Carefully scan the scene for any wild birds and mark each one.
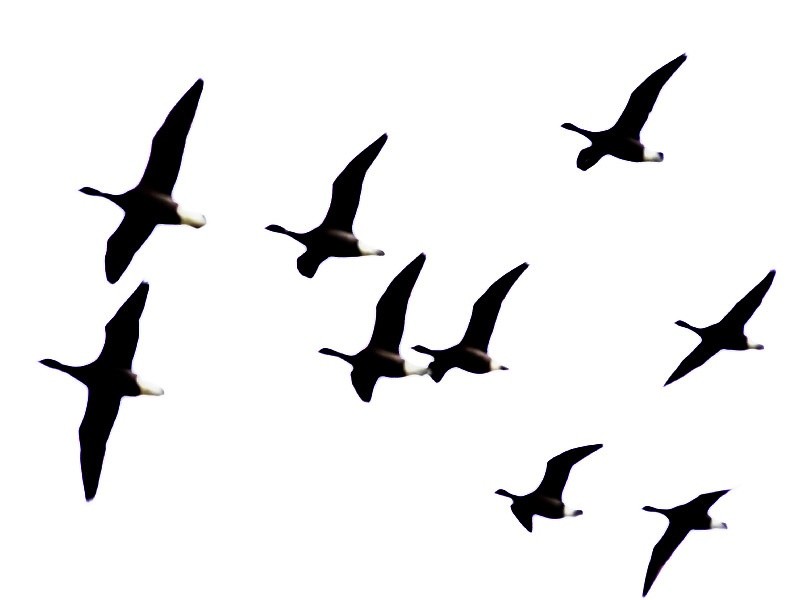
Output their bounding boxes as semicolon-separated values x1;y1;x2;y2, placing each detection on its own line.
316;252;427;401
78;77;206;283
495;444;603;531
665;269;778;385
644;490;728;597
265;134;388;280
562;52;689;171
33;283;162;502
412;260;530;382
